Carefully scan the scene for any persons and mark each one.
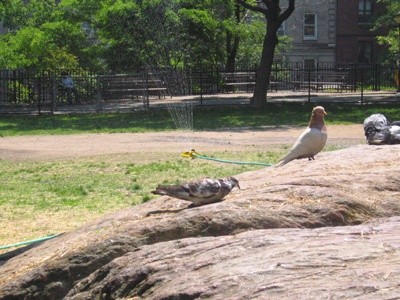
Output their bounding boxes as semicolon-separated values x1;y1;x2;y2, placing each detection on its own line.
57;70;84;107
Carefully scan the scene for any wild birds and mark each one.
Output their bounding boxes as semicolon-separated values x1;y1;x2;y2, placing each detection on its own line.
363;112;400;145
275;106;328;168
60;72;76;91
151;177;241;208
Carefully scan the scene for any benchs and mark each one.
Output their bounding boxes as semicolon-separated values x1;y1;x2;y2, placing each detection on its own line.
105;79;166;96
292;73;348;91
220;71;278;93
